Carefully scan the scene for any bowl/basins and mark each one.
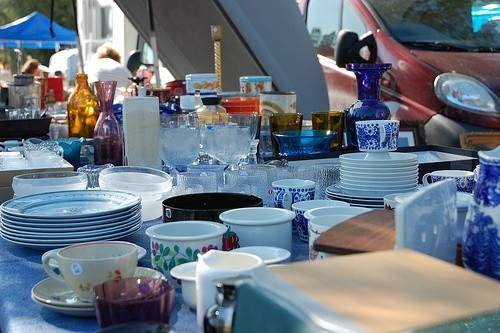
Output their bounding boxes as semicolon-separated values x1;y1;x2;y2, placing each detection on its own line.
12;171;88;193
99;166;172;224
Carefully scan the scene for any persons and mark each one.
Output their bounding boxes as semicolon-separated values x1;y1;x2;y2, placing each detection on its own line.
0;60;11;80
82;43;134;97
136;65;153;87
23;60;50;80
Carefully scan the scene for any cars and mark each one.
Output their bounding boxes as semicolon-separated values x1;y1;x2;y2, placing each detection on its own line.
295;0;500;152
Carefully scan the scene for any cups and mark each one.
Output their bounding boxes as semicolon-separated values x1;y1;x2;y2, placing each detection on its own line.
383;193;416;211
40;240;138;302
456;192;472;245
422;170;474;194
24;73;372;332
356;119;399;153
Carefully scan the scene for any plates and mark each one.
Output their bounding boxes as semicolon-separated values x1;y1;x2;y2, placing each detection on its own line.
32;290;172;317
0;192;142;252
31;267;165;308
323;147;421;212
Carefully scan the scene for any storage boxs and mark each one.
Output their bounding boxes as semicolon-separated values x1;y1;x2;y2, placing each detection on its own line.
266;142;478;183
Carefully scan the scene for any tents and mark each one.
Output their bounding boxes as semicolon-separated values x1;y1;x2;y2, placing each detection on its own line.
0;12;81;76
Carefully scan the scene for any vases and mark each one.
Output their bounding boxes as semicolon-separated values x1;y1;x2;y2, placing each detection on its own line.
344;62;393;146
92;80;122;164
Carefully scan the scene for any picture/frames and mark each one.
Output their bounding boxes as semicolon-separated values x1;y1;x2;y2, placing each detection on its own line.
398;121;424;146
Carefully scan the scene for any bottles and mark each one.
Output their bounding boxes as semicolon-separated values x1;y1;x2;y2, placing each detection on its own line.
9;71;122;166
462;146;500;281
346;62;393;147
197;96;227;124
